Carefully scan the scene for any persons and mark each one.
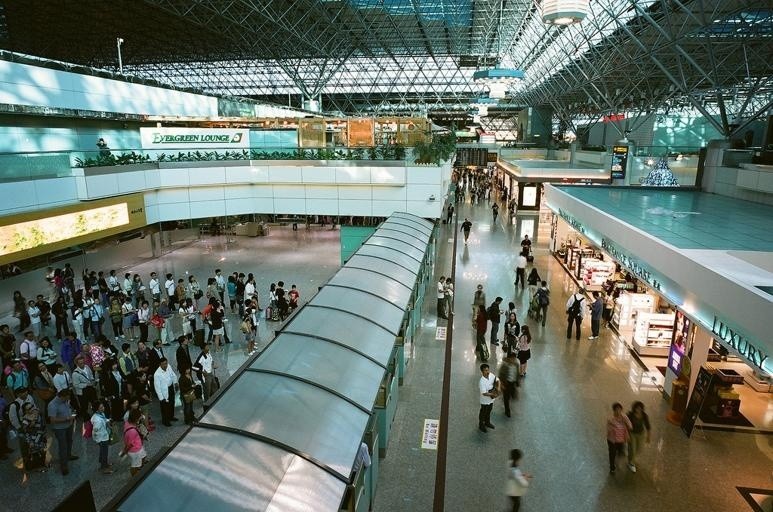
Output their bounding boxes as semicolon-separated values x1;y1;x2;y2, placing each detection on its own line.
606;402;634;475
96;138;111;157
503;449;534;512
292;215;364;231
626;401;652;473
211;217;221;236
389;132;398;144
674;311;687;353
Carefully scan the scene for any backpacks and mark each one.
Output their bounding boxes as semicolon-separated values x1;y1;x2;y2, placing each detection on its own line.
487;305;498;319
476;336;489;360
539;290;549;306
568;294;584;316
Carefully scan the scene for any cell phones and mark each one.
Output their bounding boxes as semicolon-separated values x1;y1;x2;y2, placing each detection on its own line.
71;413;77;417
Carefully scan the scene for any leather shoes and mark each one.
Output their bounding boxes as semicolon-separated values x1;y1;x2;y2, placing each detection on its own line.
162;416;179;426
480;425;487;432
70;456;79;460
486;422;494;429
505;412;511;417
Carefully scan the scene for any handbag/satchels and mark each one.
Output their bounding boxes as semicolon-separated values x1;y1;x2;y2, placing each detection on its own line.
489;377;501;399
82;419;93;439
78;386;97;401
240;316;252;333
2;369;55;421
109;426;120;445
505;467;527;497
194;290;203;299
99;311;142;326
184;389;196;403
182;316;192;336
25;448;46;470
151;316;165;328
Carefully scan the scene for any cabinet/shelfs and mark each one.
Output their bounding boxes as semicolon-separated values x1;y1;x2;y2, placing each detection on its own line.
564;246;676;355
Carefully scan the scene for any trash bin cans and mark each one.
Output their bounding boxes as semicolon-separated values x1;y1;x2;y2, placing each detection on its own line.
666;377;689;426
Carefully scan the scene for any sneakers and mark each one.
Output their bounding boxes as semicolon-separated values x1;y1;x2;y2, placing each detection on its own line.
589;336;598;340
491;339;499;346
207;340;232;353
115;335;139;342
628;463;637;472
247;342;259;356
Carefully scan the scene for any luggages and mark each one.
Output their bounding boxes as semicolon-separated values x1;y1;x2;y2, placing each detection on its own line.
266;306;281;321
527;251;534;262
194;322;205;345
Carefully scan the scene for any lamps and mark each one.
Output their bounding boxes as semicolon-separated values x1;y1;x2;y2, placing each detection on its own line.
541;0;588;25
465;1;525;133
